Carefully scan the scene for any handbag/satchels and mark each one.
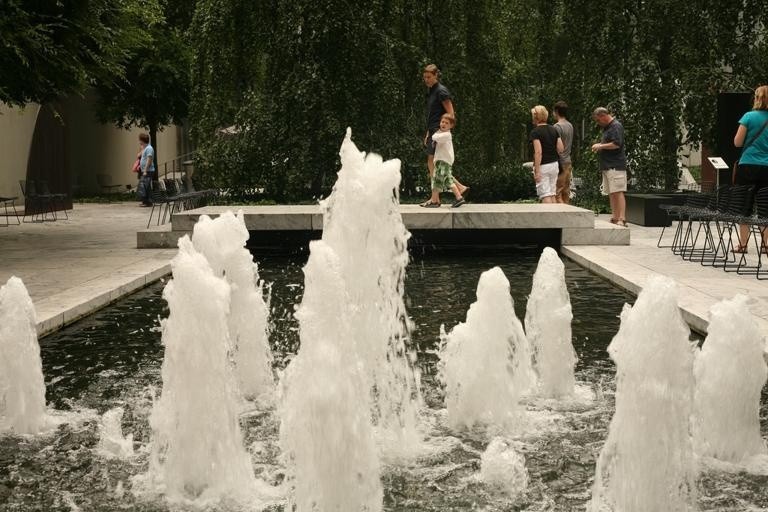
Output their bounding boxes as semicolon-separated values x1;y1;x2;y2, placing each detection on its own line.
137;176;150;198
133;160;140;171
731;160;739;186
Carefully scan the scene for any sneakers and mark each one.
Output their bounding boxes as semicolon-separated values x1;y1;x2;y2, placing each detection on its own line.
420;187;470;207
140;203;152;207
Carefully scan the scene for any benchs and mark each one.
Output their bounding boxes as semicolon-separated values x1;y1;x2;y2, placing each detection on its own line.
619;192;688;228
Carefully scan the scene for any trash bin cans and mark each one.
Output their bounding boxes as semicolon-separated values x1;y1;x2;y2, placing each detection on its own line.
182;160;196;193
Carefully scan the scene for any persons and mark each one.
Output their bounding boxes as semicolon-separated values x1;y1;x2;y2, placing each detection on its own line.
731;85;768;253
530;104;565;203
591;107;627;227
550;100;574;205
423;113;466;208
137;133;156;207
137;143;155;203
419;63;471;206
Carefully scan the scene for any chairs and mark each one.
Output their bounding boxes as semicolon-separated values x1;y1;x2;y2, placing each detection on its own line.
94;171;124;204
0;176;71;229
143;172;222;236
651;179;767;286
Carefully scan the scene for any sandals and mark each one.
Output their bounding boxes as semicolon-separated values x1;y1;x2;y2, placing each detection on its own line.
731;244;747;253
